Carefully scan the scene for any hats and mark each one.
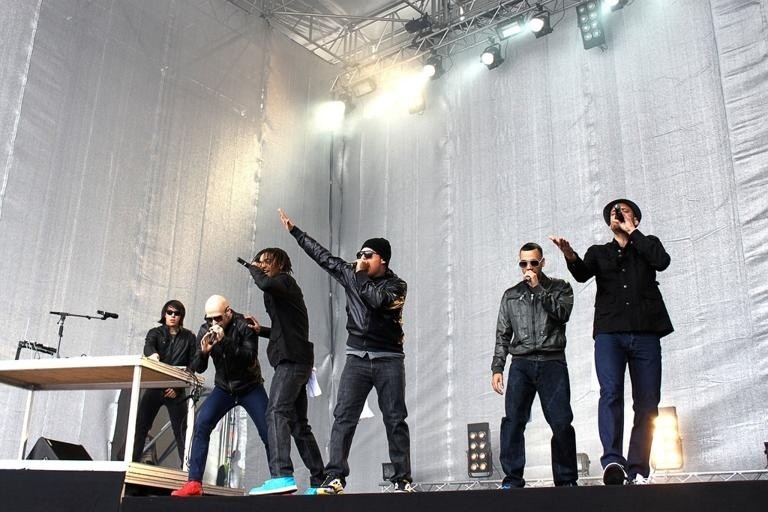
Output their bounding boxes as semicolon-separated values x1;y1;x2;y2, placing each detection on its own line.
362;236;392;263
602;198;643;228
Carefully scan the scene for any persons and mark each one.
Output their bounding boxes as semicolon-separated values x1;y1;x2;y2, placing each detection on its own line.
547;199;669;486
277;206;412;495
129;299;196;471
247;247;325;497
490;242;577;488
169;295;269;497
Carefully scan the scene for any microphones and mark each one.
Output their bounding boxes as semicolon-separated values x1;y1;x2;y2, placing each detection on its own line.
344;260;368;269
613;203;627;225
236;256;263;272
97;309;118;318
208;323;222;345
524;271;534;282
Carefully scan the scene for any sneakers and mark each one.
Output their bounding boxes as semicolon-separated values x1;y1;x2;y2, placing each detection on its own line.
600;463;627;487
303;486;318;496
502;482;517;489
170;480;204;498
315;471;345;494
626;472;651;484
391;478;413;493
247;476;299;496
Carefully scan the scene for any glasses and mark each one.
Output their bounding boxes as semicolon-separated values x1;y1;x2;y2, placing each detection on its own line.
518;257;545;268
166;309;183;317
356;250;378;260
203;313;226;323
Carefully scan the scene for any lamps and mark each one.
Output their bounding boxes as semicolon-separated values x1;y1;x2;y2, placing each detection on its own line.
602;461;630;486
575;1;605;51
336;93;357;118
530;11;553;38
423;57;445;82
610;0;630;13
576;454;591;478
467;422;493;477
653;406;685;471
494;16;527;42
382;462;397;481
0;355;203;507
351;80;377;98
400;70;427;117
480;43;505;72
